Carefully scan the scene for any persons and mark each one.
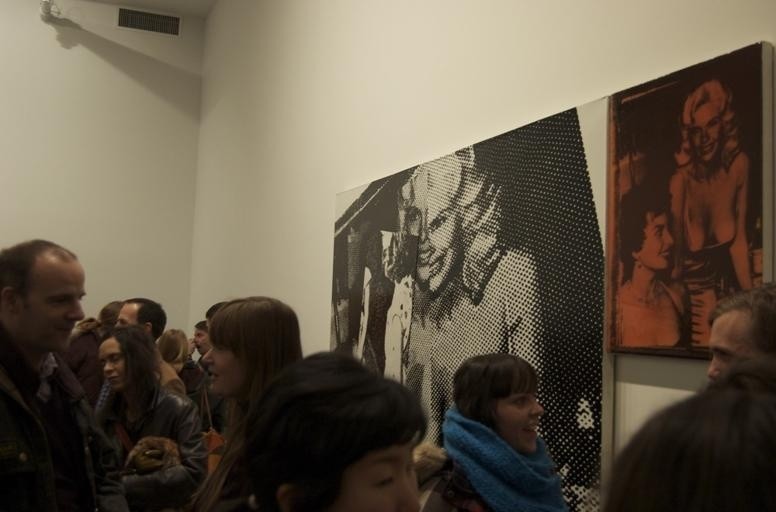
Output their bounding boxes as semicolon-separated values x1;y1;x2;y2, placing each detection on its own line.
1;239;426;512
614;173;690;348
600;352;776;509
707;283;775;383
669;78;754;299
431;142;548;453
382;163;430;462
417;353;572;512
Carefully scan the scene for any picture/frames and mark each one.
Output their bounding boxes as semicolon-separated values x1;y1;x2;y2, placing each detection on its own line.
607;40;772;360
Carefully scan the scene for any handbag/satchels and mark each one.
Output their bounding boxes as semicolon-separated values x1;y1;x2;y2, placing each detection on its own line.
203;427;225;476
